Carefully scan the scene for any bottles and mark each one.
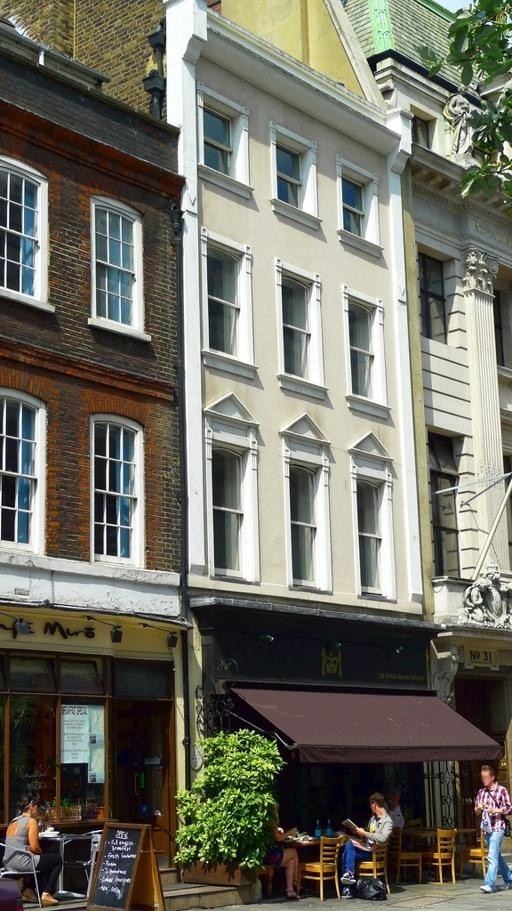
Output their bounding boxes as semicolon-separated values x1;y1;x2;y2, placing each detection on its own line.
326;819;334;837
314;818;322;840
38;819;44;832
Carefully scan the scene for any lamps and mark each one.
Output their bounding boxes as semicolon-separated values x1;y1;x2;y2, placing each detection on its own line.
81;614;123;644
1;612;30;636
138;623;178;649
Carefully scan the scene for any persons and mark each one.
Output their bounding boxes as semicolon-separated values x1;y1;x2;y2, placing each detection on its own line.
466;577;489;622
261;826;306;901
383;792;406;885
339;792;394;901
460;564;508;627
472;764;512;894
2;790;64;908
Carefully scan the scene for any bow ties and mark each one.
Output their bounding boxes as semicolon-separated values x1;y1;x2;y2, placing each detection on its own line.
374;815;381;820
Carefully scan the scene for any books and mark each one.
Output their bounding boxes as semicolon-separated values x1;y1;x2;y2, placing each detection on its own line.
341;819;358;833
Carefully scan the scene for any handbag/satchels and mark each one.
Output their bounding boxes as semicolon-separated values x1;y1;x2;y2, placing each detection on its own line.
504;818;511;837
352;878;387;900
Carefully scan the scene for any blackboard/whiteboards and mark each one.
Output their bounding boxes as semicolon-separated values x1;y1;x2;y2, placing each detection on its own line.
86;822;148;911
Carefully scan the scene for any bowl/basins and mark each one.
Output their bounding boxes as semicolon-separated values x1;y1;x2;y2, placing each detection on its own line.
38;827;60;837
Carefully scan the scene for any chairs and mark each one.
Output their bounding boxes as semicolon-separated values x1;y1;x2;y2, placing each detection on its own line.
0;843;46;909
258;819;497;903
62;830;104;893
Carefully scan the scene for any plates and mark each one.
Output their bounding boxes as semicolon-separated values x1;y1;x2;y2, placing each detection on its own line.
282;830;313;844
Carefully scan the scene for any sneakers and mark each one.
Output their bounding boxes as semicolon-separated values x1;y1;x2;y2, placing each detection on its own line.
341;871;356;885
342;886;352;898
480;884;497;893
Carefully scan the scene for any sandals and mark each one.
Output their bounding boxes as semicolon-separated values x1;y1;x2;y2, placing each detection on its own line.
285;889;303;899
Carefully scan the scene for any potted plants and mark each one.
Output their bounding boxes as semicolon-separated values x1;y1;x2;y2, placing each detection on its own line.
43;797;84;823
175;729;284;887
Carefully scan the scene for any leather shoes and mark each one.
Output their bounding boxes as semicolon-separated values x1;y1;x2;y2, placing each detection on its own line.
22;896;39;903
40;896;59;907
